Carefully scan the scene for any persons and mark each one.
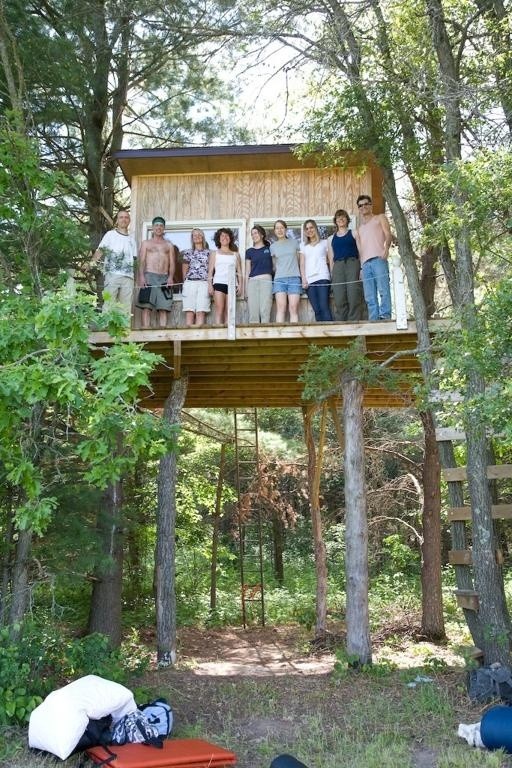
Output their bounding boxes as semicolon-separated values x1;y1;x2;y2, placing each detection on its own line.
83;210;137;331
136;217;175;329
182;228;210;325
327;209;363;321
269;219;304;323
298;220;332;322
244;225;273;323
355;195;391;320
208;227;242;324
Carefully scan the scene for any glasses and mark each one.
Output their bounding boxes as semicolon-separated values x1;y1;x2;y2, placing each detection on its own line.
359;202;369;208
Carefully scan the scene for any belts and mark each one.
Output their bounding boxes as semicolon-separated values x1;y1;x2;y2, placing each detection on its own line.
185;277;206;281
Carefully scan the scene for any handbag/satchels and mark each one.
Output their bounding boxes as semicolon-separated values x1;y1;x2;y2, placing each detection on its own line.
142;698;173;736
28;714;112;761
